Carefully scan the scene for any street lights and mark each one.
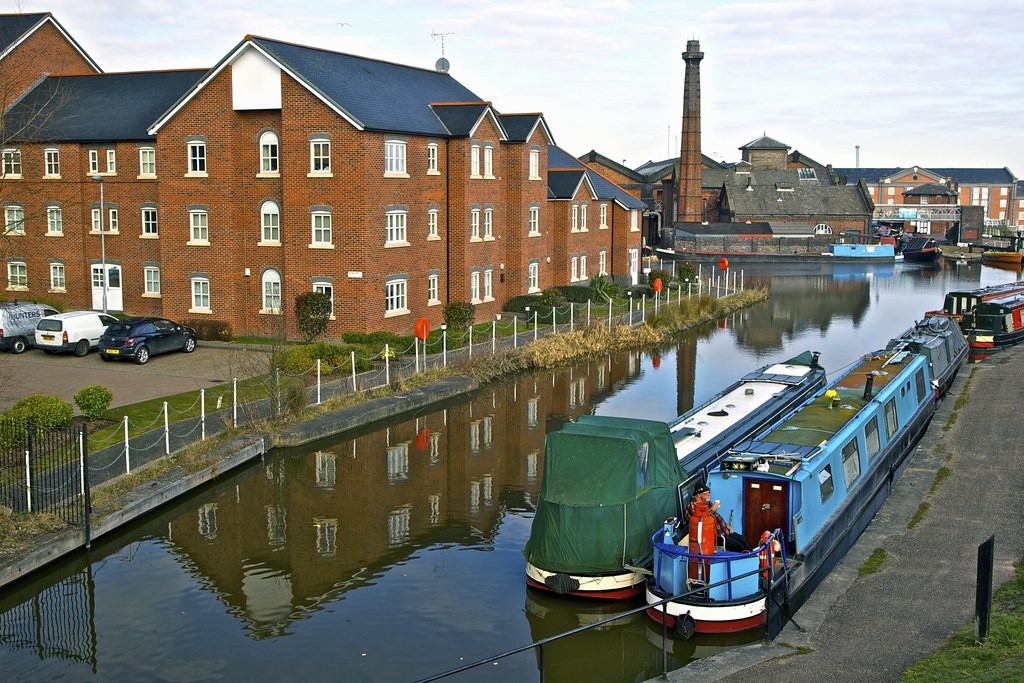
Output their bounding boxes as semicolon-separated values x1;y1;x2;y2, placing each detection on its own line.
91;175;108;319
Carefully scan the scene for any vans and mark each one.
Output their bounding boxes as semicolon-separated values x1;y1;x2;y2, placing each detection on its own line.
34;310;120;357
0;299;62;354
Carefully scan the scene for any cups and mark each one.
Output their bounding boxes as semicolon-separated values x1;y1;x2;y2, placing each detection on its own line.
715;500;720;508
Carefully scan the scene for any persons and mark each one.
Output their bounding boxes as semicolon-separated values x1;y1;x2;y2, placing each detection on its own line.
685;482;752;552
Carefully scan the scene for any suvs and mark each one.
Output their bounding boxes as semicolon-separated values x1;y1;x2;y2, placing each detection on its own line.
97;316;198;365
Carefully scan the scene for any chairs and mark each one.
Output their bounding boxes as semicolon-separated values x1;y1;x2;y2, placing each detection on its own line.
685;501;727;553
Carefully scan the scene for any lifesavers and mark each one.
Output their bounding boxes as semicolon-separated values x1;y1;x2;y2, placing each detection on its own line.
759;529;777;579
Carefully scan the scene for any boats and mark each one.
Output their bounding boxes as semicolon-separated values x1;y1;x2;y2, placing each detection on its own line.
983;250;1022;263
903;238;941;261
645;349;935;639
522;349;828;602
886;280;1024;397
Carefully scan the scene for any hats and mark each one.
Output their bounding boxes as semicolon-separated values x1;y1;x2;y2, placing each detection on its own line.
693;484;709;495
